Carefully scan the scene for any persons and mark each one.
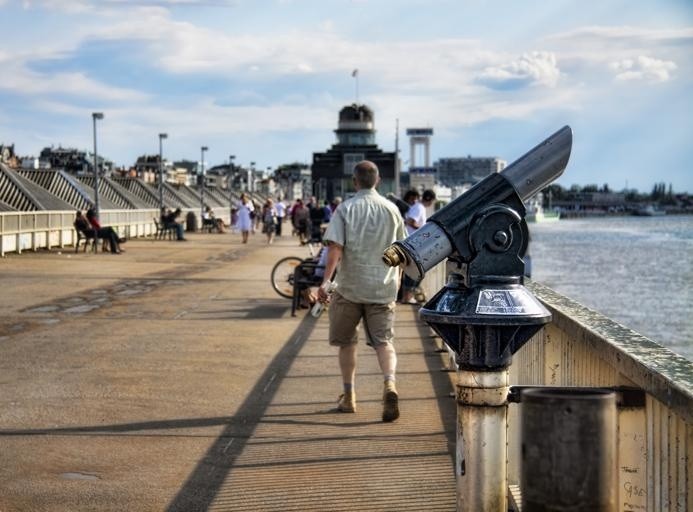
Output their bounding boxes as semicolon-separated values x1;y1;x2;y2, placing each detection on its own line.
154;184;437;313
74;210;126;254
85;207;126;252
316;159;410;423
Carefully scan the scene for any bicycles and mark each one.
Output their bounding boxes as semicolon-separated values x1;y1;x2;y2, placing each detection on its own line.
271;254;311;298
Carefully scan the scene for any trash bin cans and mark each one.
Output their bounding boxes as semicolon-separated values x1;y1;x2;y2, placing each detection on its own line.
186;212;196;232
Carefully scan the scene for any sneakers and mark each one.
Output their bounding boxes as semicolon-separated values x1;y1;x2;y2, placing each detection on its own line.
401;298;420;305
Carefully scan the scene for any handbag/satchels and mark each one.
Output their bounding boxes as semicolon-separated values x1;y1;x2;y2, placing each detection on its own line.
299;218;306;225
271;217;278;224
249;211;256;219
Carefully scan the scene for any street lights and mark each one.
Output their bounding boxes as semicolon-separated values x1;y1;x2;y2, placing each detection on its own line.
249;161;254;202
200;147;209;229
158;133;167;213
228;155;235;207
91;112;103;223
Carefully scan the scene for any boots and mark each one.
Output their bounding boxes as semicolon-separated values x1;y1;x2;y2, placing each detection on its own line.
337;389;356;413
382;380;400;422
